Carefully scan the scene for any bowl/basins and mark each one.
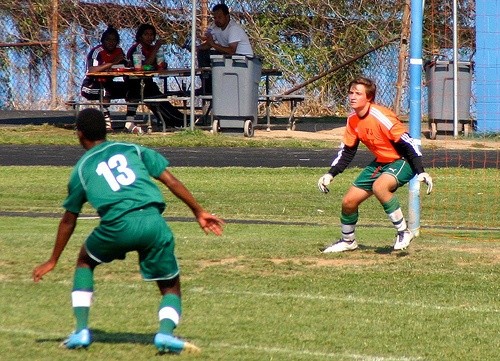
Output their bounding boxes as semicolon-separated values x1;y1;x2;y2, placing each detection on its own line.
111;65;124;71
143;65;154;71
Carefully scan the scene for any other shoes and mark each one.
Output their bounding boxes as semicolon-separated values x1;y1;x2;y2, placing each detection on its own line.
124;123;143;133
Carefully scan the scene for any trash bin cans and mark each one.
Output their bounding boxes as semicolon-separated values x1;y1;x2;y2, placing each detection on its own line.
209;53;264;137
423;60;473;140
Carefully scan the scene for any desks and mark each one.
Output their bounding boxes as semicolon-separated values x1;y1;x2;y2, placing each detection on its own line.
85;67;283;134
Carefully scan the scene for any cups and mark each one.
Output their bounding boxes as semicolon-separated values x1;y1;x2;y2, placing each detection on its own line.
133;52;142;70
156;52;165;70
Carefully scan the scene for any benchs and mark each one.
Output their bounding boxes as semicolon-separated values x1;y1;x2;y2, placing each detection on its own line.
63;89;306;132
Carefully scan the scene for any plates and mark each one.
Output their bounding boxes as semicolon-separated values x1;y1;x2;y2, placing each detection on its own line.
124;67;134;71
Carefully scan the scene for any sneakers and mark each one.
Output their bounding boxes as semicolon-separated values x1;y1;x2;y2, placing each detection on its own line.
394;228;414;251
324;239;357;253
61;329;90;348
154;333;201;355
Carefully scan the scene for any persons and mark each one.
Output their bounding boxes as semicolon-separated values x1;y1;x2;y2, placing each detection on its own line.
81;29;144;134
197;3;254;96
32;108;226;355
318;77;433;253
125;23;199;128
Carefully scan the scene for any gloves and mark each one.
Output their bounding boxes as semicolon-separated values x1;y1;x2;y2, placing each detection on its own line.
318;174;334;194
417;173;432;195
